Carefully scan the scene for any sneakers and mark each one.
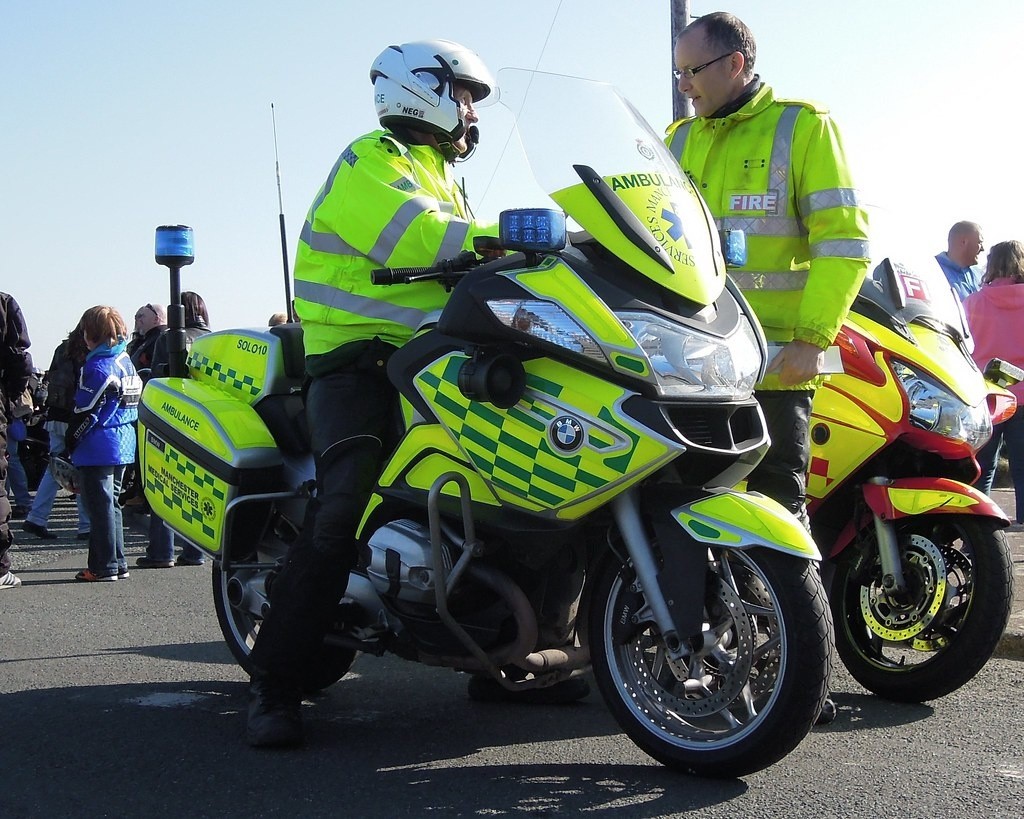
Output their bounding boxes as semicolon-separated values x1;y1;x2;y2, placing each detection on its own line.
245;663;303;749
74;562;130;582
0;571;21;588
135;549;203;567
468;656;590;706
22;519;60;542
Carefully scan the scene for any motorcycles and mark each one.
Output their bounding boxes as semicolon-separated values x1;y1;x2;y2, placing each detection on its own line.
807;258;1024;701
131;66;837;780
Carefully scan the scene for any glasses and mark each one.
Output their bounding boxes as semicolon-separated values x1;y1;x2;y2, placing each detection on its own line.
674;53;731;80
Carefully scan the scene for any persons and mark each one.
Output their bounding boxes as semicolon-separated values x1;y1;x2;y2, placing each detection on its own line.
935;223;984;299
0;276;210;590
663;12;870;723
244;39;588;749
962;242;1024;522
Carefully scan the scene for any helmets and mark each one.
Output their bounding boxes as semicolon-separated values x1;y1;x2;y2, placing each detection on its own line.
371;41;492;139
